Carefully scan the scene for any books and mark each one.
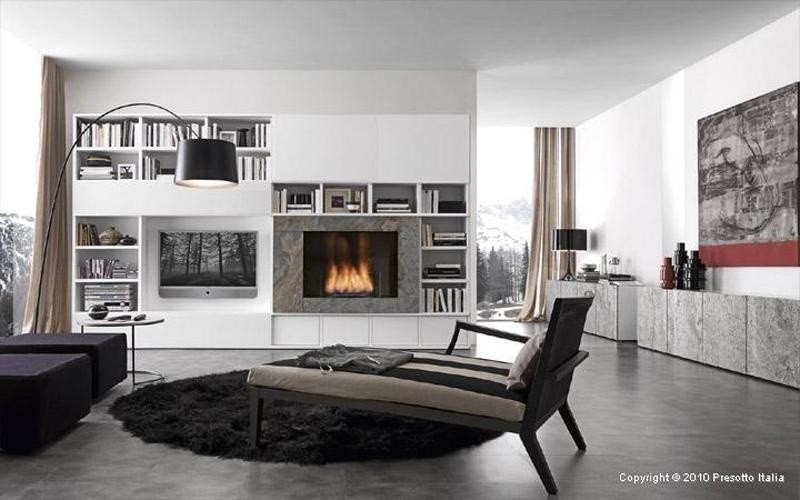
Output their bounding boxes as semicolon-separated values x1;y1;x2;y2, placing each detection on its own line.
272;182;466;215
578;270;645;288
74;117;269;184
77;221;137;311
421;223;468;314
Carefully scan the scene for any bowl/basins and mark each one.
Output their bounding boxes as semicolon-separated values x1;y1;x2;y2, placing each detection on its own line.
580;264;597;272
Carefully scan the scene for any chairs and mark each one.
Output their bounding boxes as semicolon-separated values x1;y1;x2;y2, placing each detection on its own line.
245;291;594;496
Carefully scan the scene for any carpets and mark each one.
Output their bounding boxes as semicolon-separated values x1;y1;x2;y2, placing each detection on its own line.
105;358;506;467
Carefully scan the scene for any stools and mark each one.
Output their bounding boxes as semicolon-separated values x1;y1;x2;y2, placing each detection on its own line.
1;333;128;456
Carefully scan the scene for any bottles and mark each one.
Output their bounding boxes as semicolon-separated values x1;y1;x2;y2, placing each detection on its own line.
600;254;607;279
609;257;620;274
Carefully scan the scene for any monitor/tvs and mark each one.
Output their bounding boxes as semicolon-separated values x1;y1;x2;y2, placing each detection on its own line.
158;229;257;298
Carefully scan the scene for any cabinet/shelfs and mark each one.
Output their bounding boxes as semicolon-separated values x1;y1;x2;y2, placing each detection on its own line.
544;278;800;391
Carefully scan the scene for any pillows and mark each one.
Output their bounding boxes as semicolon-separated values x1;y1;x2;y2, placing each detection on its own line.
504;329;548;393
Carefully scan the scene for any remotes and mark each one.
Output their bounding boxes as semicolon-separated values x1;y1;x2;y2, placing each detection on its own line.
135;314;145;320
109;315;131;321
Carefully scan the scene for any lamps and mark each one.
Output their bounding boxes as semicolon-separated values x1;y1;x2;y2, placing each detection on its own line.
551;228;587;281
33;102;238;333
